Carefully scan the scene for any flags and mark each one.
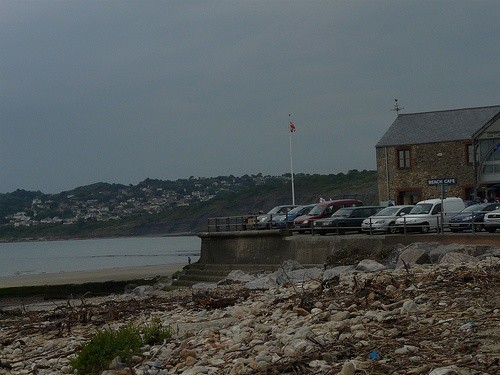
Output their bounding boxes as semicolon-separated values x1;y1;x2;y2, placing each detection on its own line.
290;122;296;133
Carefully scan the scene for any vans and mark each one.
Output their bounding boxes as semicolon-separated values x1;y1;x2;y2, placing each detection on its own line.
395;196;466;233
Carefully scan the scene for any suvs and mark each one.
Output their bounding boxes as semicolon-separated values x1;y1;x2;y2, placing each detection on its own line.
293;198;364;234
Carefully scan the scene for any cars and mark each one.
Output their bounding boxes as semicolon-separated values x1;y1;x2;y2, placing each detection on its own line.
448;202;500;232
252;204;300;230
483;207;500;233
313;206;387;235
276;204;316;229
361;204;415;234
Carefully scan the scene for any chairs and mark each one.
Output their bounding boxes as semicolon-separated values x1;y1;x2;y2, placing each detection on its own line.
347;211;356;217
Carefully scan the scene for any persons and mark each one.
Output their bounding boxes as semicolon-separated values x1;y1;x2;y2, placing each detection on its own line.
479;195;500;203
